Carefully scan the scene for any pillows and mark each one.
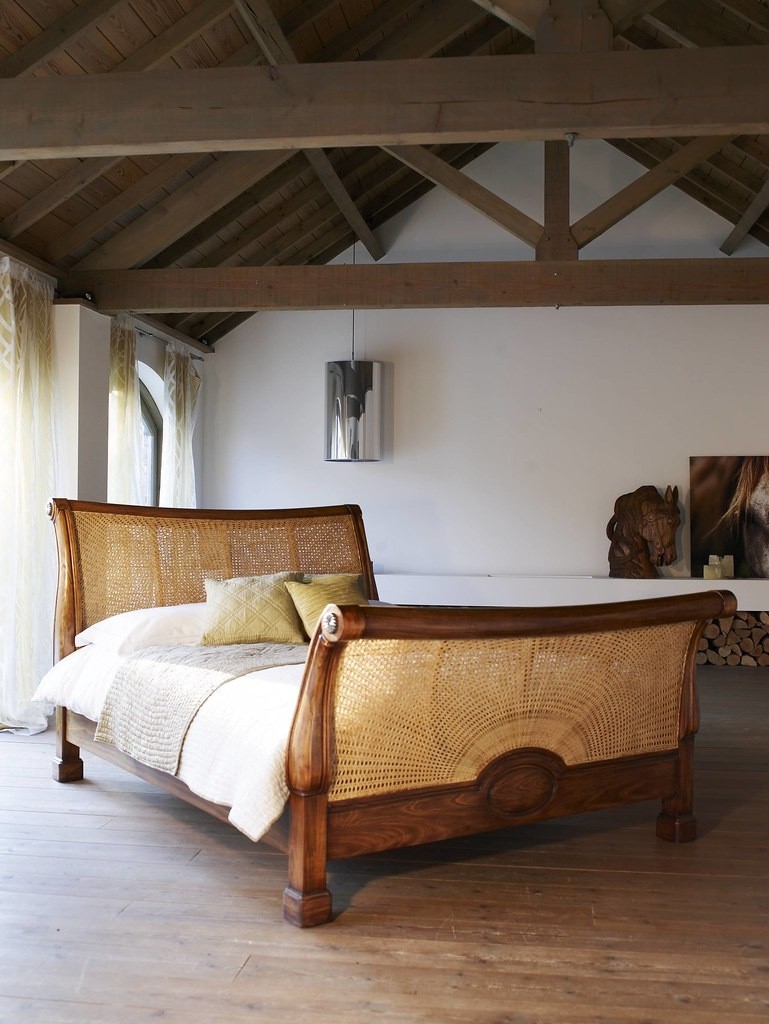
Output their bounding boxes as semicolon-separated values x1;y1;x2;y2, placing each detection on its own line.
75;602;206;661
282;574;370;638
202;570;306;645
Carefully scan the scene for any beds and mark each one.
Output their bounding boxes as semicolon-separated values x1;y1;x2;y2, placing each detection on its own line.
29;497;739;930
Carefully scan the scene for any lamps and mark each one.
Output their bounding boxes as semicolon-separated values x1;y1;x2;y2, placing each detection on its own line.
322;237;379;462
